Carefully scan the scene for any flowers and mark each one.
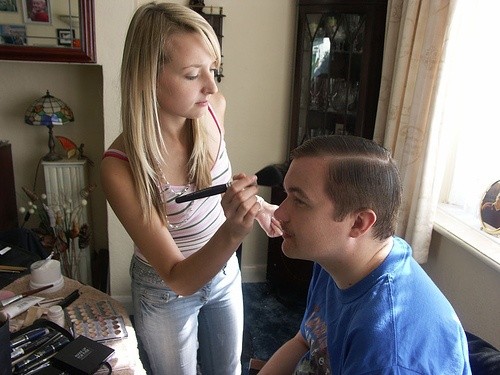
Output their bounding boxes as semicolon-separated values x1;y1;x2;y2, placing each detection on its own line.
12;187;92;250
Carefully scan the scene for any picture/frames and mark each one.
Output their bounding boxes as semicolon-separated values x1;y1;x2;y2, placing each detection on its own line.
0;0;97;64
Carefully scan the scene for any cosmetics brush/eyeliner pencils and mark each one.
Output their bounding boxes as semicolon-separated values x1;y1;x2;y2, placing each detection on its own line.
1;284;56;309
10;327;70;372
176;165;281;204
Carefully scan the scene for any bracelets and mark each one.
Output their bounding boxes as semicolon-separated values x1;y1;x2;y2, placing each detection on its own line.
256;196;263;215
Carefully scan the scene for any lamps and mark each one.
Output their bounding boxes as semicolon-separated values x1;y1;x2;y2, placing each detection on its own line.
25;89;74;161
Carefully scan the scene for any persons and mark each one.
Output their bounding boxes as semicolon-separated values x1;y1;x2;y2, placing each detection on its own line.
258;134;473;375
101;2;284;375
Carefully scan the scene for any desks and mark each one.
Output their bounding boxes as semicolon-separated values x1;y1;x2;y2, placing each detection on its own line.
0;274;148;375
42;158;93;286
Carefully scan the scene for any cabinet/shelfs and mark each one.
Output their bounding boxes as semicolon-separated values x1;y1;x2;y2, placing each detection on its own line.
264;0;387;298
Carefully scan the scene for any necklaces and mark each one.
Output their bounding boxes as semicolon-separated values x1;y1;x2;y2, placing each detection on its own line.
150;152;200;229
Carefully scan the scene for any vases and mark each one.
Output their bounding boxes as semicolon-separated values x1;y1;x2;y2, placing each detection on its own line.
60;232;82;282
